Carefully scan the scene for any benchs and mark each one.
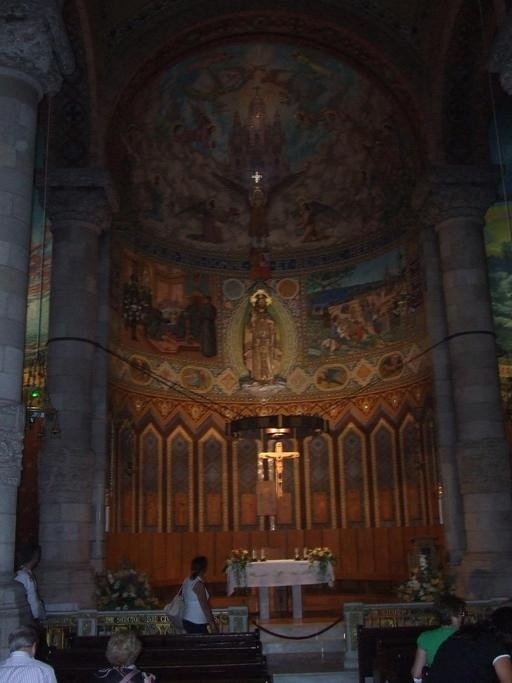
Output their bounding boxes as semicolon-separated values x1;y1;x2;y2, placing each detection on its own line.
357;624;440;683
36;629;275;683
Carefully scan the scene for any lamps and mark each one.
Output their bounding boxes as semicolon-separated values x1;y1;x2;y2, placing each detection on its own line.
224;413;330;498
23;95;61;437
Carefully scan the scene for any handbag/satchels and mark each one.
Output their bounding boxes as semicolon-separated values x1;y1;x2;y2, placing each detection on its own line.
163;581;188;630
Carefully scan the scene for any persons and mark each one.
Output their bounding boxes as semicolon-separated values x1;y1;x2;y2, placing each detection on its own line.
409;593;468;682
197;294;218;356
87;627;158;682
320;295;390;357
179;554;220;634
1;625;60;683
13;539;48;620
240;294;277;382
426;605;511;682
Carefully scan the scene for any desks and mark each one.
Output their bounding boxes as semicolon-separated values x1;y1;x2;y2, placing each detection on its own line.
225;559;335;620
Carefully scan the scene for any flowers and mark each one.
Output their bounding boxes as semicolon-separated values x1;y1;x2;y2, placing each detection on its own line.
308;547;334;575
224;547;249;595
96;559;159;610
393;552;457;602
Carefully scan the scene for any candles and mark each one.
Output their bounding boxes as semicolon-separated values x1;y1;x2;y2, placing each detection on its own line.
261;549;264;559
295;546;299;558
304;547;307;558
253;549;256;560
